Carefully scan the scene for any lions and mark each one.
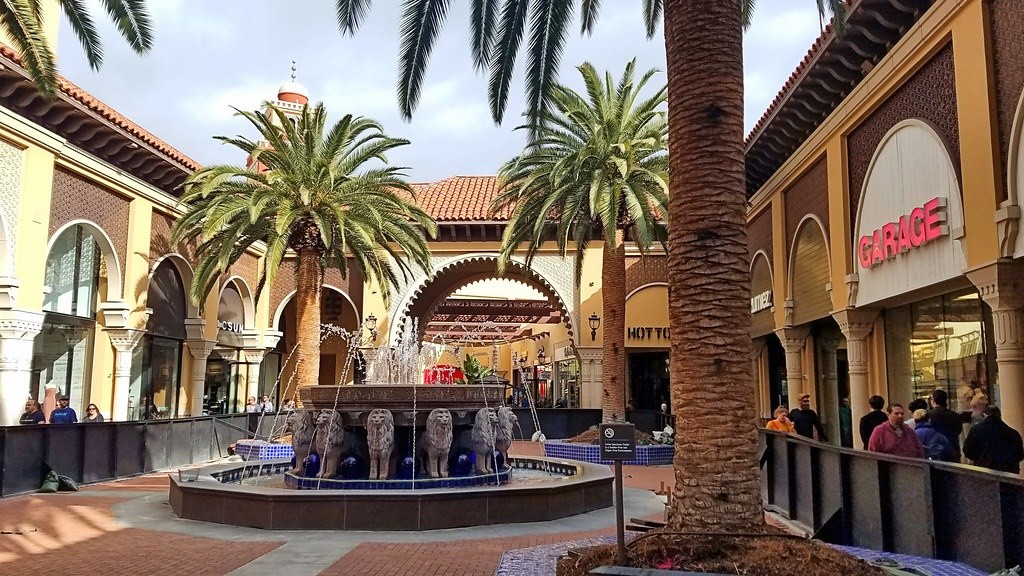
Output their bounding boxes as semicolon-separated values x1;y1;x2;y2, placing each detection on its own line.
287;408;518;480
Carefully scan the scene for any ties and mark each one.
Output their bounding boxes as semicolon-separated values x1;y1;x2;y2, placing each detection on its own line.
262;404;265;411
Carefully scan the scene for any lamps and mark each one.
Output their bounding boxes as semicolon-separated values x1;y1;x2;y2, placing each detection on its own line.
588;312;601;341
365;312;377;341
161;367;170;381
537;345;545;364
519;355;528;367
664;353;670;373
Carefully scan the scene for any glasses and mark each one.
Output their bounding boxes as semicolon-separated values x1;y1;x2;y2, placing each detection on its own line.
88;408;95;411
800;400;809;403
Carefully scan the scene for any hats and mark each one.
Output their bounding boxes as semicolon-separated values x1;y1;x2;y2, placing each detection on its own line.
797;394;811;401
59;395;69;400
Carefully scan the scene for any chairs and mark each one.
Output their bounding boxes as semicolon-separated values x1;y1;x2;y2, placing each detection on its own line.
203;399;210;412
208;400;224;415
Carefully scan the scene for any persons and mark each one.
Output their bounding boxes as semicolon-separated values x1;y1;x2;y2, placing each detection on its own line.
82;404;104;422
242;396;261;413
282;398;294;408
507;393;564;408
259;394;272;412
49;395;77;424
787;394;828;442
766;406;793;434
859;390;1024;474
838;397;852;447
19;400;45;425
